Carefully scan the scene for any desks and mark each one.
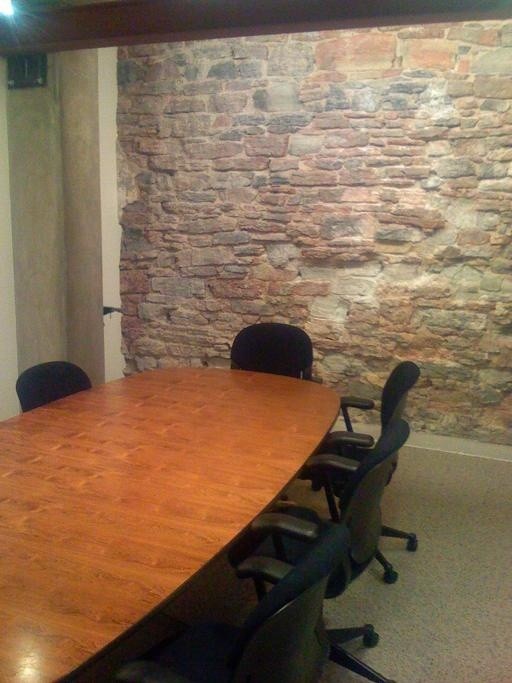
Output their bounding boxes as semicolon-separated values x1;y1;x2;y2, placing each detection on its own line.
0;362;344;682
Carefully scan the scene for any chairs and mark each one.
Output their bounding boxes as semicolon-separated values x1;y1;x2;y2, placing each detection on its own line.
273;413;419;586
296;359;422;493
231;447;397;683
13;360;93;413
230;319;317;381
105;520;355;681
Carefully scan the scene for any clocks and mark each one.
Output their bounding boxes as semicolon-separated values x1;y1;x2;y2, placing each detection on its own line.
6;51;46;90
